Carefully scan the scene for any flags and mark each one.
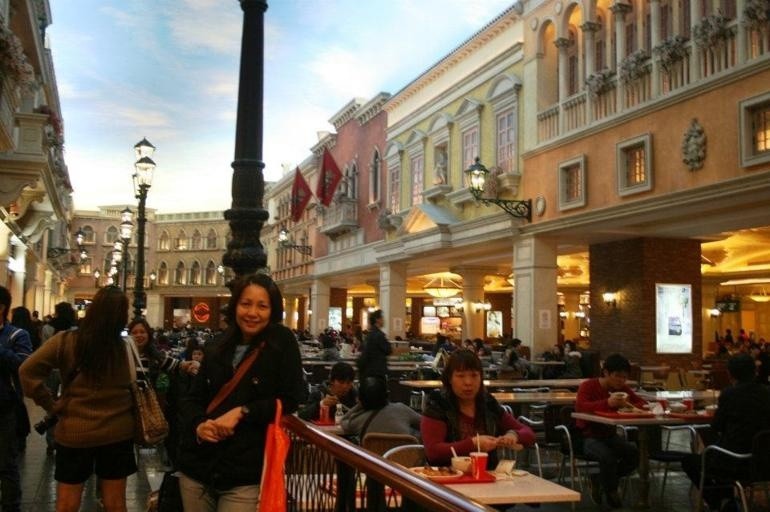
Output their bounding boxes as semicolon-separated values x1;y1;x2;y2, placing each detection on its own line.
316;148;343;207
291;166;313;223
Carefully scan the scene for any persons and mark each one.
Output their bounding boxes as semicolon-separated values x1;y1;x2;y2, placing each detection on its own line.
567;350;599;377
0;285;33;512
298;362;359;419
748;331;755;343
357;310;392;380
473;338;485;356
346;324;352;338
564;340;575;353
182;304;229;396
680;352;770;512
31;310;41;327
339;375;422;446
176;339;198;361
574;354;648;510
420;347;535;471
19;283;137;512
498;346;522;378
750;344;761;366
356;326;363;342
318;334;343;361
431;335;447;357
12;306;36;351
172;272;308;512
740;328;748;339
509;339;522;351
758;342;770;384
127;317;200;391
553;344;564;357
44;315;52;323
726;329;732;343
759;338;765;351
40;301;78;455
446;335;455;346
191;344;204;363
464;339;474;352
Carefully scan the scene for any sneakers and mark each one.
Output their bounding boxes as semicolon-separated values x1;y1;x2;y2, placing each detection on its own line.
589;477;602;507
607;492;622;509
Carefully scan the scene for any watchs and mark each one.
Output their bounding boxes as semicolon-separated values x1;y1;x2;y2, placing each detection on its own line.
240;404;250;417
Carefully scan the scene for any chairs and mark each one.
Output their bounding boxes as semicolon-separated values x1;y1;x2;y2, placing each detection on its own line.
360;433;421;458
382;445;431;469
511;388;550;469
697;431;769;512
544;403;627;511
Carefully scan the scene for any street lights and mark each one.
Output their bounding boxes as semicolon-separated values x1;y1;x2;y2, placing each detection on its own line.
112;239;122;286
131;137;156;323
118;205;135;294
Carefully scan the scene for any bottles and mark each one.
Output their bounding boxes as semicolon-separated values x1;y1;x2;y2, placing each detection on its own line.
334;403;345;433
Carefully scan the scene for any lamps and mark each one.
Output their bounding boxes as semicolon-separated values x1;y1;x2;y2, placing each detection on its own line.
423;275;463;298
603;280;617;303
747;286;770;303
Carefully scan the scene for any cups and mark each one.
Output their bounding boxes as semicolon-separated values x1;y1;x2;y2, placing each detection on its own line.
705;405;717;417
451;457;471;473
469;452;488;480
683;399;694;410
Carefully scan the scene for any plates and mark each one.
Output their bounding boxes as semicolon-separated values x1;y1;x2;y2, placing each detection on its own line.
593;409;672;419
410;466;463;479
432;471;496;485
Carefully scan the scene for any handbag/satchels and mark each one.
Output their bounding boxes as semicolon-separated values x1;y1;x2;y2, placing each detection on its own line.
256;399;291;512
125;335;169;448
